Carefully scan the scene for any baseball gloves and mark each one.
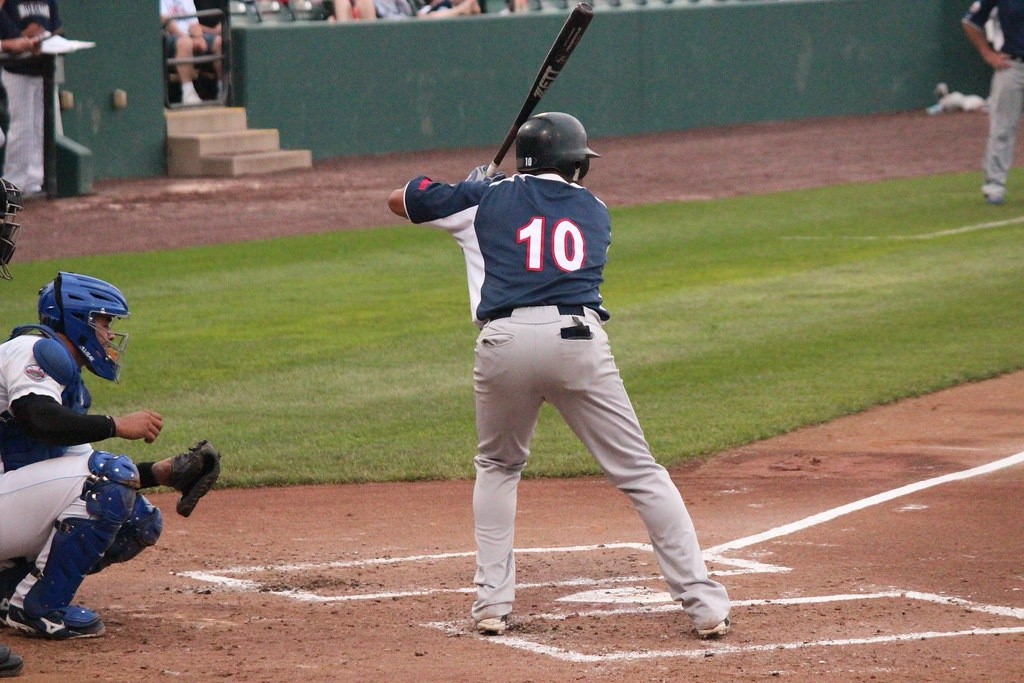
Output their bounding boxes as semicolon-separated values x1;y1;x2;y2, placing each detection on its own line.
169;440;221;519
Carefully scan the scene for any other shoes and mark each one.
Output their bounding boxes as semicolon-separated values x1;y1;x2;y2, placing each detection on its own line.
478;615;505;634
698;620;730;640
983;185;1004;206
183;93;204;105
0;645;23;678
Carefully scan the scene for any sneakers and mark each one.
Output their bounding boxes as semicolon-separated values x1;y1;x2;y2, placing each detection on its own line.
7;601;106;641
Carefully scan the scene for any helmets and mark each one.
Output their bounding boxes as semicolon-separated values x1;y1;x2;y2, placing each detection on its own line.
40;269;130;381
515;111;601;181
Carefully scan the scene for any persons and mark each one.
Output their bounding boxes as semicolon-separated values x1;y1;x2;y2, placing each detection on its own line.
0;271;221;641
159;0;228;105
389;112;733;639
0;0;63;678
321;0;529;23
960;0;1024;205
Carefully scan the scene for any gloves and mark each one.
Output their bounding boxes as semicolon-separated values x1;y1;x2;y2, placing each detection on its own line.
465;167;505;186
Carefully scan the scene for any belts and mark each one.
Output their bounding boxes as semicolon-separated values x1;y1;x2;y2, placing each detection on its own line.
1009;55;1024;64
490;305;586;323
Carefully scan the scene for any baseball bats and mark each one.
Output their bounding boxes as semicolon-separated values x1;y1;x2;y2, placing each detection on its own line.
483;2;596;177
